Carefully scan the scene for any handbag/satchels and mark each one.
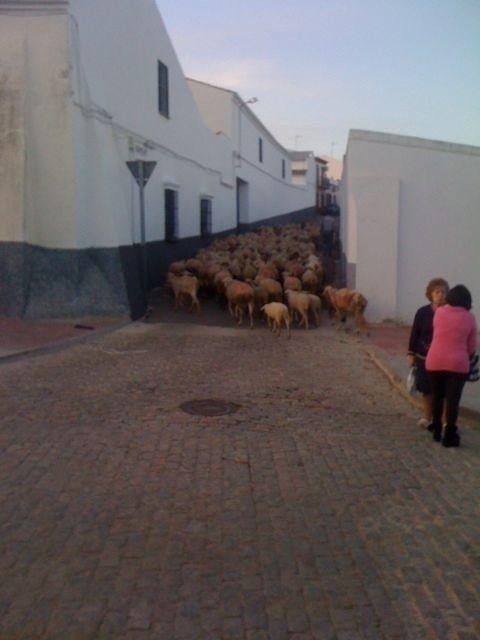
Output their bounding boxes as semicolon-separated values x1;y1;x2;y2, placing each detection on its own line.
406;367;419;396
467;351;480;382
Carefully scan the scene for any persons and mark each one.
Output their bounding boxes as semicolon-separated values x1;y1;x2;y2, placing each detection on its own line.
425;284;477;448
320;209;340;252
406;278;449;429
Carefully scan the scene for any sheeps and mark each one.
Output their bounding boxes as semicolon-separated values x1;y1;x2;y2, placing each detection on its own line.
260;301;291;339
164;219;324;329
320;285;370;337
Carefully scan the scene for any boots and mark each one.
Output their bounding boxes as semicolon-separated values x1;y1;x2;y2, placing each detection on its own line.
416;418;460;447
